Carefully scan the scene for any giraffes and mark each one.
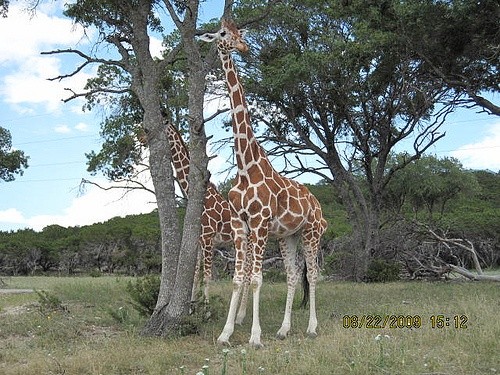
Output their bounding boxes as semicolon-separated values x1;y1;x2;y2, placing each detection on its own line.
138;109;255;326
192;18;328;351
